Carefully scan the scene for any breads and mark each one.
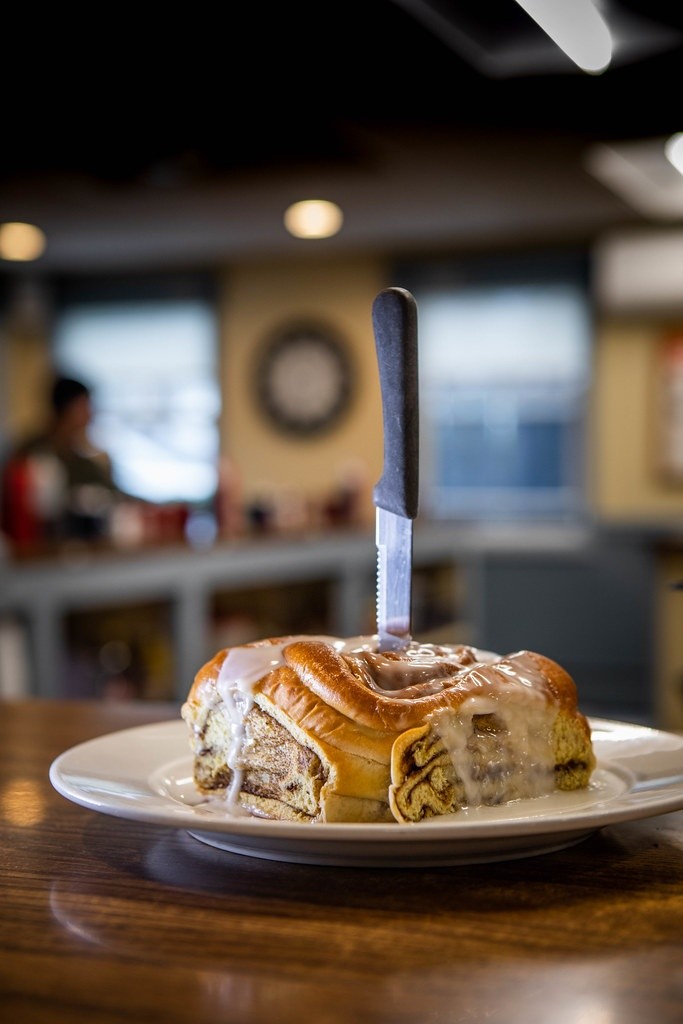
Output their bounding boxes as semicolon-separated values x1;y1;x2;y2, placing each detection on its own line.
180;631;595;821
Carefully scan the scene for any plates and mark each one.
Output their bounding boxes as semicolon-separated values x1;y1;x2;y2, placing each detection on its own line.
49;715;683;868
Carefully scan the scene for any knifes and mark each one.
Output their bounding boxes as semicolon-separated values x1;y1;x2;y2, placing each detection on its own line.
371;284;420;655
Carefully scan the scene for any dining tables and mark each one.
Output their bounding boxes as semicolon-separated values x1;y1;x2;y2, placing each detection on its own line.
1;693;683;1024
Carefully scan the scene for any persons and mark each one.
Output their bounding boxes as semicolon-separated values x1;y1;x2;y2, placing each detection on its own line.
0;379;117;696
